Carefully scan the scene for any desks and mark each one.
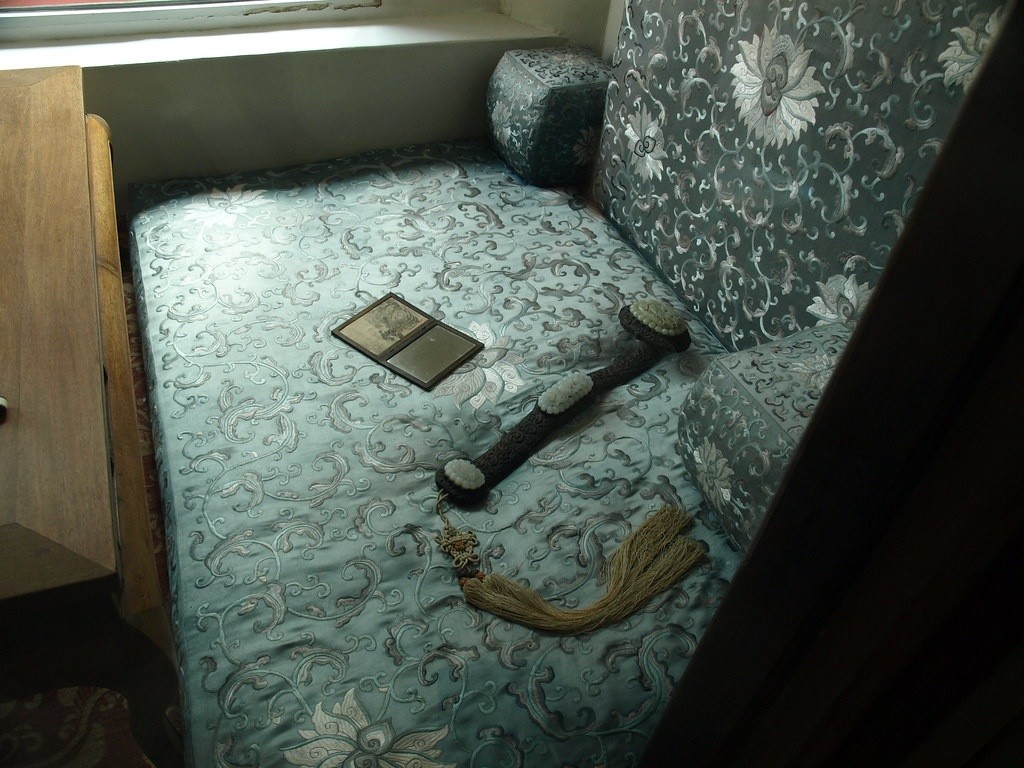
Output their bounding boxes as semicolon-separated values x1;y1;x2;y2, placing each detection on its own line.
0;66;174;662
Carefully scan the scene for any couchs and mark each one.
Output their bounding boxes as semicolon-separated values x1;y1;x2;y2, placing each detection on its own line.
126;0;1024;768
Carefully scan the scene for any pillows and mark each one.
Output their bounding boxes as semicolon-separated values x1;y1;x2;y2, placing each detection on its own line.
676;323;850;551
486;40;612;183
